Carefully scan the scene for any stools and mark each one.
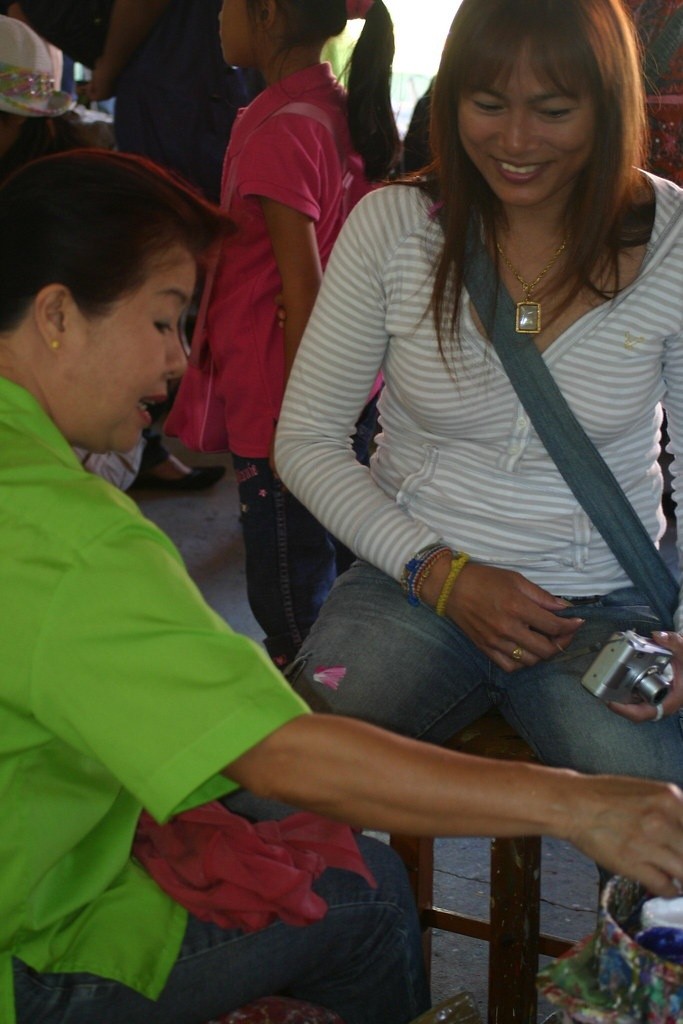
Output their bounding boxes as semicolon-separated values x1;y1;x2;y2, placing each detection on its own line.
388;737;613;1023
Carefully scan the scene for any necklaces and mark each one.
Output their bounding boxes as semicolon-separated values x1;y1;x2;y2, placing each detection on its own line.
493;224;572;335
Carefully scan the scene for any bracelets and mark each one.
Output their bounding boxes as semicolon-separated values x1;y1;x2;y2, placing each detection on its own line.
437;551;468;617
402;543;451;607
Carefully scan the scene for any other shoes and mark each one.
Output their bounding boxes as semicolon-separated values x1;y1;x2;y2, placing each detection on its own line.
130;461;226;490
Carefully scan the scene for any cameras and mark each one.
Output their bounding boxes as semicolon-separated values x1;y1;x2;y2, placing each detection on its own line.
581;630;673;706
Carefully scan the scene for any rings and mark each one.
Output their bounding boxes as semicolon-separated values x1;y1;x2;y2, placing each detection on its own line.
512;646;523;661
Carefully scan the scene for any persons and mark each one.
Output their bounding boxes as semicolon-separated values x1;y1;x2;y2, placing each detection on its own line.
0;144;683;1024
160;0;403;669
383;0;683;187
0;0;265;494
271;0;683;937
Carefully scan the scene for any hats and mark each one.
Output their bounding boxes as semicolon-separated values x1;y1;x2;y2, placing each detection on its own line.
0;15;75;116
